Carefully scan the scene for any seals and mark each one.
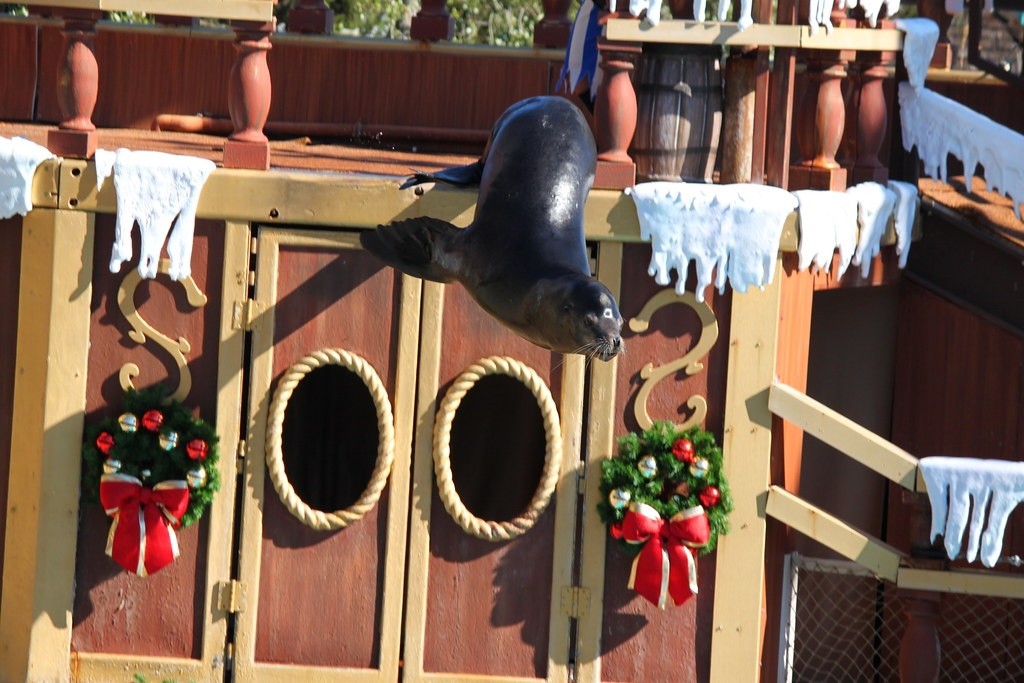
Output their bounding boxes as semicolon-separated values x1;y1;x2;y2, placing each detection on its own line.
357;95;627;365
